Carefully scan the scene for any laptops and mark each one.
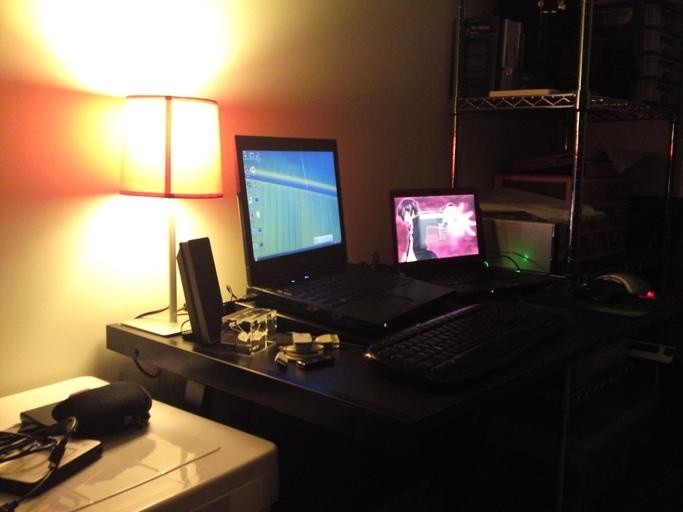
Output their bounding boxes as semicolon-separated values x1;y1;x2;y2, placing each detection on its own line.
235;135;455;336
388;188;551;302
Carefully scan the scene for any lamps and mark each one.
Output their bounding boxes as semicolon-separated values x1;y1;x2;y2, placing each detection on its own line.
120;94;226;341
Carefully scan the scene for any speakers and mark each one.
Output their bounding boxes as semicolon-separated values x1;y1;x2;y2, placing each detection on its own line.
177;237;226;344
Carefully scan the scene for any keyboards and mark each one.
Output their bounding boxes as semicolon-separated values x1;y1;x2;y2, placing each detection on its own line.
366;300;567;383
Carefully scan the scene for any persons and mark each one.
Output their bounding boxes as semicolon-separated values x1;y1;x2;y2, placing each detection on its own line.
398;200;477;263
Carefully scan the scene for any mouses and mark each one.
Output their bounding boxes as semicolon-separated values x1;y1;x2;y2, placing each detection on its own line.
583;271;656;304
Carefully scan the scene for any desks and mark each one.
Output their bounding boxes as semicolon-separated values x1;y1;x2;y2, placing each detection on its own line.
106;278;682;511
1;373;282;510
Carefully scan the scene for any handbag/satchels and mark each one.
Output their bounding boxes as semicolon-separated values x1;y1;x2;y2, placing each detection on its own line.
52;381;152;434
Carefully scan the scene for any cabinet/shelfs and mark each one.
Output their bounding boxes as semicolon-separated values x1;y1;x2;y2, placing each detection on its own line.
445;0;683;300
